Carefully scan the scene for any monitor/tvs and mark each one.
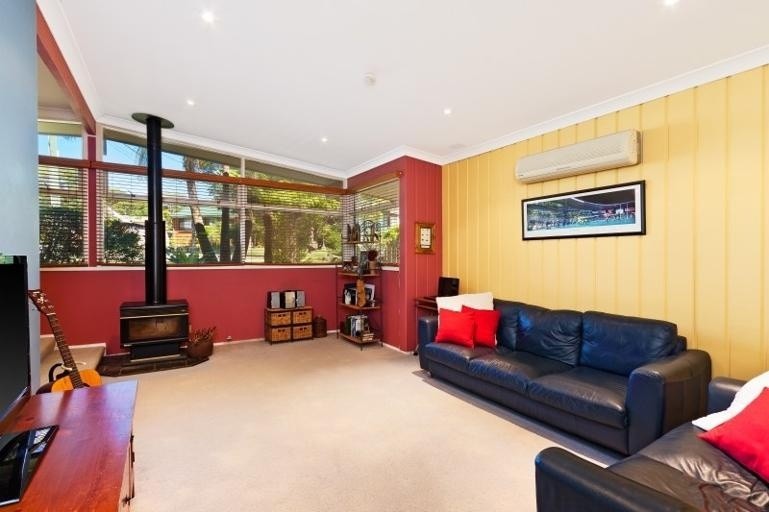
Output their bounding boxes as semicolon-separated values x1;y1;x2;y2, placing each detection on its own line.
0;254;60;507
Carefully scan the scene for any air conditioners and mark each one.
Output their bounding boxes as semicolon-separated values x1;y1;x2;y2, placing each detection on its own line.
514;129;640;185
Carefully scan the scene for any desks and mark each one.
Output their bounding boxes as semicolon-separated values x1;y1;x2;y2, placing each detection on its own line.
414;295;437;355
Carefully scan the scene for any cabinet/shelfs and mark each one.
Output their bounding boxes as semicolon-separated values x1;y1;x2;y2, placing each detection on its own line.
264;304;313;345
1;378;139;512
335;220;383;351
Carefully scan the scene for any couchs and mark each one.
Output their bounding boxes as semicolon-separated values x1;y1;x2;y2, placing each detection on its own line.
417;297;713;456
534;375;769;512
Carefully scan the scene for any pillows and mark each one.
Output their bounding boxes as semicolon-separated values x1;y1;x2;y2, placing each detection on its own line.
697;386;769;485
435;307;475;349
435;292;498;345
460;304;500;349
691;370;769;432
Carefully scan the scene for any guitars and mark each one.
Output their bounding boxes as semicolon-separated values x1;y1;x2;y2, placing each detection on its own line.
28;289;104;394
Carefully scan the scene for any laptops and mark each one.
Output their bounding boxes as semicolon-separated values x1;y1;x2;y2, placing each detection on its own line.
424;276;460;301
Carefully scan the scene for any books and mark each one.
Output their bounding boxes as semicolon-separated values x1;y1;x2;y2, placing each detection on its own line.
346;314;374;342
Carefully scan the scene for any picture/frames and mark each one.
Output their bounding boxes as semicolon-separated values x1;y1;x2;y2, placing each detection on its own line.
414;221;437;255
521;180;646;240
